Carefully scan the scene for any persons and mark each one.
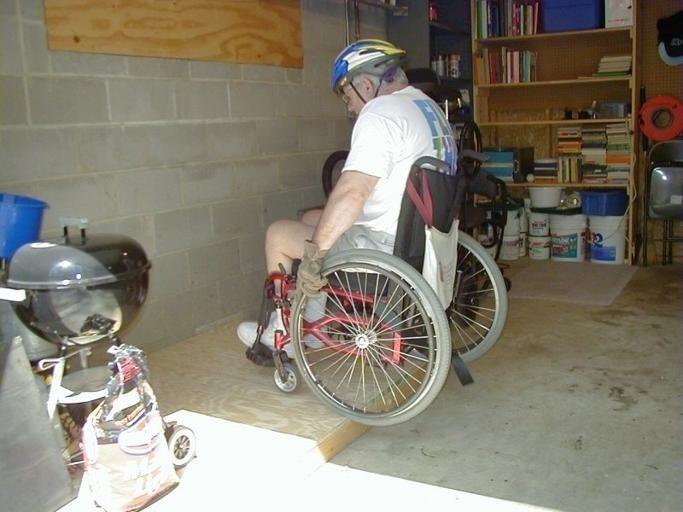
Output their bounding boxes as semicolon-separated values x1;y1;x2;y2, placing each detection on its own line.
234;36;460;363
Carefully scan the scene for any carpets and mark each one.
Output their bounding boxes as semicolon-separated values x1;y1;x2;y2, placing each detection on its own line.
486;261;640;307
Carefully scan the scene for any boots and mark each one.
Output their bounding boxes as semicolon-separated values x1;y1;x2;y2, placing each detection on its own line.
237;290;328;360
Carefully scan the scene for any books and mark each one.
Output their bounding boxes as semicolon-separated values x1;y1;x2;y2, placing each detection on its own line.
473;2;634;187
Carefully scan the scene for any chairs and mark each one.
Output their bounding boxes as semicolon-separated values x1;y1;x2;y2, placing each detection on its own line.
646;140;683;265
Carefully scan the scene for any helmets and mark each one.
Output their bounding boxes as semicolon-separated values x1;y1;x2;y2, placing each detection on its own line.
332;37;407;95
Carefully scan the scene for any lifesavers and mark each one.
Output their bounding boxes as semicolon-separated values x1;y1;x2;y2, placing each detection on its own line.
639;96;682;141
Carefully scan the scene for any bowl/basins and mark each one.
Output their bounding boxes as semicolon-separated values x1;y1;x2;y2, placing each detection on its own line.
524;186;565;208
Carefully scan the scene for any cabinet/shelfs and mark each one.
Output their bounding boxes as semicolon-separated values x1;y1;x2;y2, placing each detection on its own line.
386;0;470;127
470;0;642;265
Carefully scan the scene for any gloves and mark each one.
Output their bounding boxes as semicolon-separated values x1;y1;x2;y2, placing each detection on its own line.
295;239;330;298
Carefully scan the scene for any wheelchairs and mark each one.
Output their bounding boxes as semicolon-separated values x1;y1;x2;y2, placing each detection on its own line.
246;120;509;429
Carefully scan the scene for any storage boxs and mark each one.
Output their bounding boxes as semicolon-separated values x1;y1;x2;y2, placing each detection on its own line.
541;0;600;32
605;0;634;29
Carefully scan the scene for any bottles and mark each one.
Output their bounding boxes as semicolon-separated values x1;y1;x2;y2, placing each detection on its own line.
430;54;462;78
429;3;438;21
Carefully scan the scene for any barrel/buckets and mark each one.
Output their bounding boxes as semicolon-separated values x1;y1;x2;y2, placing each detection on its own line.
528;211;550;237
528;235;552;260
549;214;588;264
498;236;521;260
587;214;627;265
502;207;522;236
